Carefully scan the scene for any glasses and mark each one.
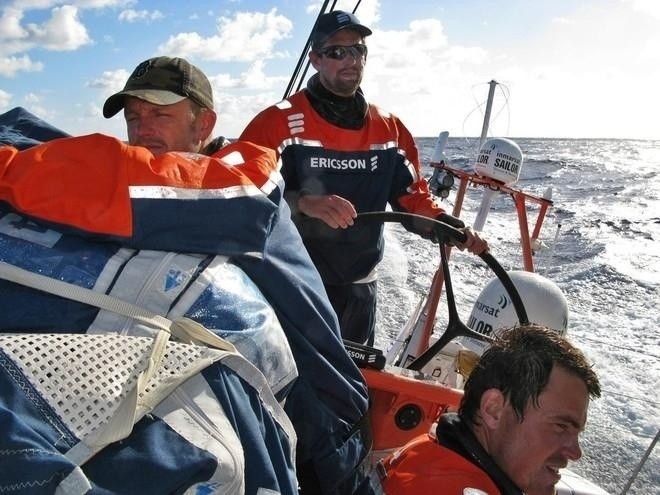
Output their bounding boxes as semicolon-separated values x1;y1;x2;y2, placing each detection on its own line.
316;45;368;60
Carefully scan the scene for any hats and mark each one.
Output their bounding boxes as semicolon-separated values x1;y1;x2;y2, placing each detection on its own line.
311;11;372;48
103;56;213;119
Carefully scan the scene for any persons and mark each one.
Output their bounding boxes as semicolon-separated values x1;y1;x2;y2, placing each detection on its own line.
352;325;600;495
1;57;370;492
238;10;491;348
